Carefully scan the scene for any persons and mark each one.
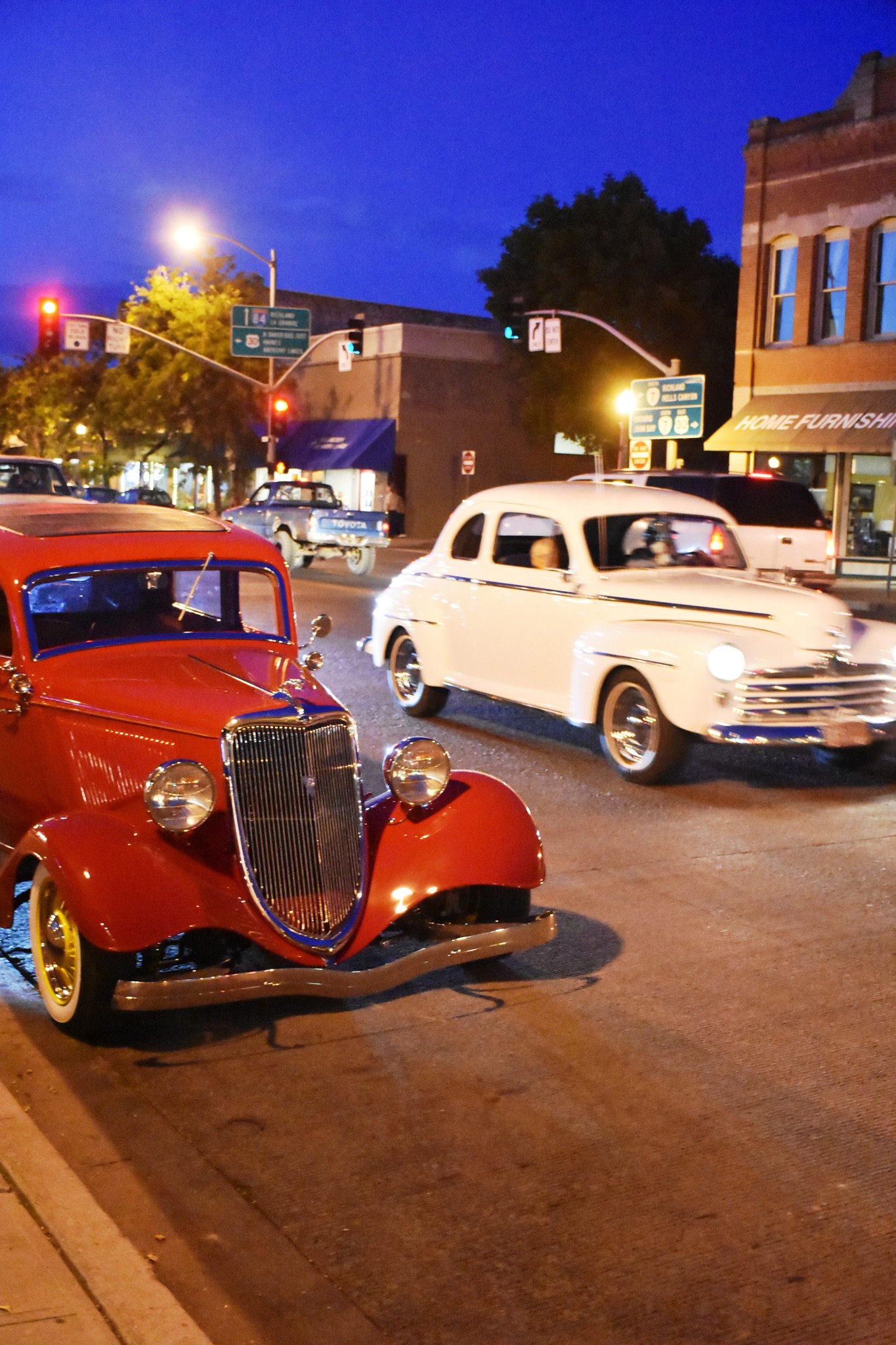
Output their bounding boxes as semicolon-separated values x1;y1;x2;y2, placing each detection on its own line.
624;517;719;567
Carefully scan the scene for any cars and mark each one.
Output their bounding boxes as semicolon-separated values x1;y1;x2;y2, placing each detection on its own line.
352;481;896;788
78;487;122;503
0;491;557;1027
117;488;175;511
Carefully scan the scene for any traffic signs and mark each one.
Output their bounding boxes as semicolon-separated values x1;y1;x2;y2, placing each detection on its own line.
628;373;706;441
231;305;311;360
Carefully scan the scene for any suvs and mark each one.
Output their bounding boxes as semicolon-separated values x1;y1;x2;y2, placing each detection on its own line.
0;454;78;499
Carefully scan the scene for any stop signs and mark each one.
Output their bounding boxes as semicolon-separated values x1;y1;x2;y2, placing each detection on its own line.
629;440;652;471
461;450;476;474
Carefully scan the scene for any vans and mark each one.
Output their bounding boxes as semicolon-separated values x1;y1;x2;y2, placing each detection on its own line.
566;470;837;594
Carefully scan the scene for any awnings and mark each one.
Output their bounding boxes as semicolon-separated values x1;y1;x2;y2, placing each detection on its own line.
697;385;895;462
272;417;396;475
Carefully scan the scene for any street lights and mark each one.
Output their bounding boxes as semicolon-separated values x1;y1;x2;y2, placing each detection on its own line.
176;221;276;479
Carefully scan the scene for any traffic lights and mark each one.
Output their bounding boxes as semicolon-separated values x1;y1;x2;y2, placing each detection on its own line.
36;296;61;363
500;293;525;341
269;396;288;436
345;312;364;356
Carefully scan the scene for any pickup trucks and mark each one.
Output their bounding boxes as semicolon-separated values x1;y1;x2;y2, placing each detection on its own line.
220;480;390;575
